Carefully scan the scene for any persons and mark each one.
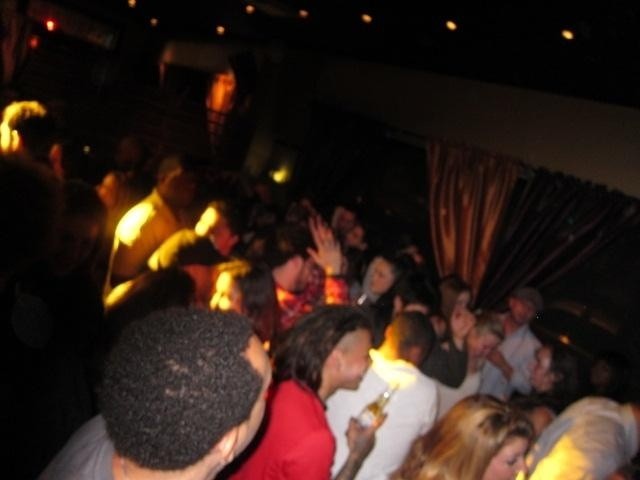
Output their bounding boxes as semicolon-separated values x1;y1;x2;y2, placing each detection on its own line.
0;99;640;480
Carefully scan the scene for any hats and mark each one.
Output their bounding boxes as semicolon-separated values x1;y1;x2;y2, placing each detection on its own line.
513;288;544;310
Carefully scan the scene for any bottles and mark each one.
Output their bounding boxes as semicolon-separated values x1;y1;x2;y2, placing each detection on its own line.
357;380;397;432
